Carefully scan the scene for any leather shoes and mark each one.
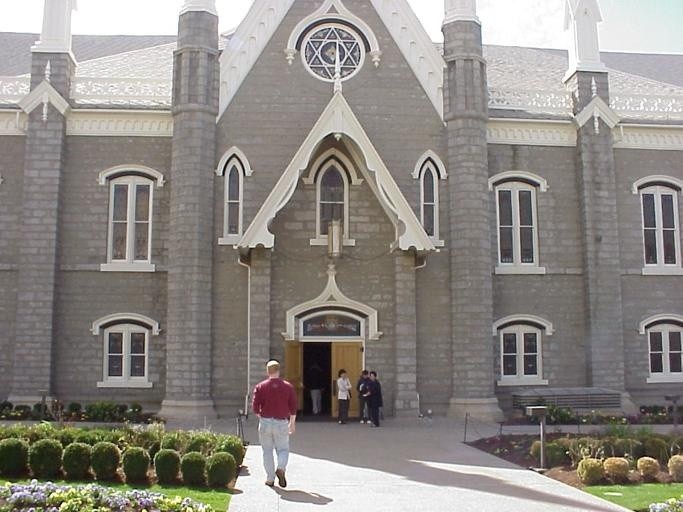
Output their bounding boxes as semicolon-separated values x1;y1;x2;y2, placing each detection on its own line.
266;481;275;487
276;469;286;488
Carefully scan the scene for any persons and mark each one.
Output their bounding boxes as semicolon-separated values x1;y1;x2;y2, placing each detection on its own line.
304;368;324;414
366;370;383;427
251;359;297;487
356;370;371;423
336;368;351;423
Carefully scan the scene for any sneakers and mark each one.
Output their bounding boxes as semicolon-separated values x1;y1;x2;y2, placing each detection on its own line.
360;420;364;424
367;420;371;424
